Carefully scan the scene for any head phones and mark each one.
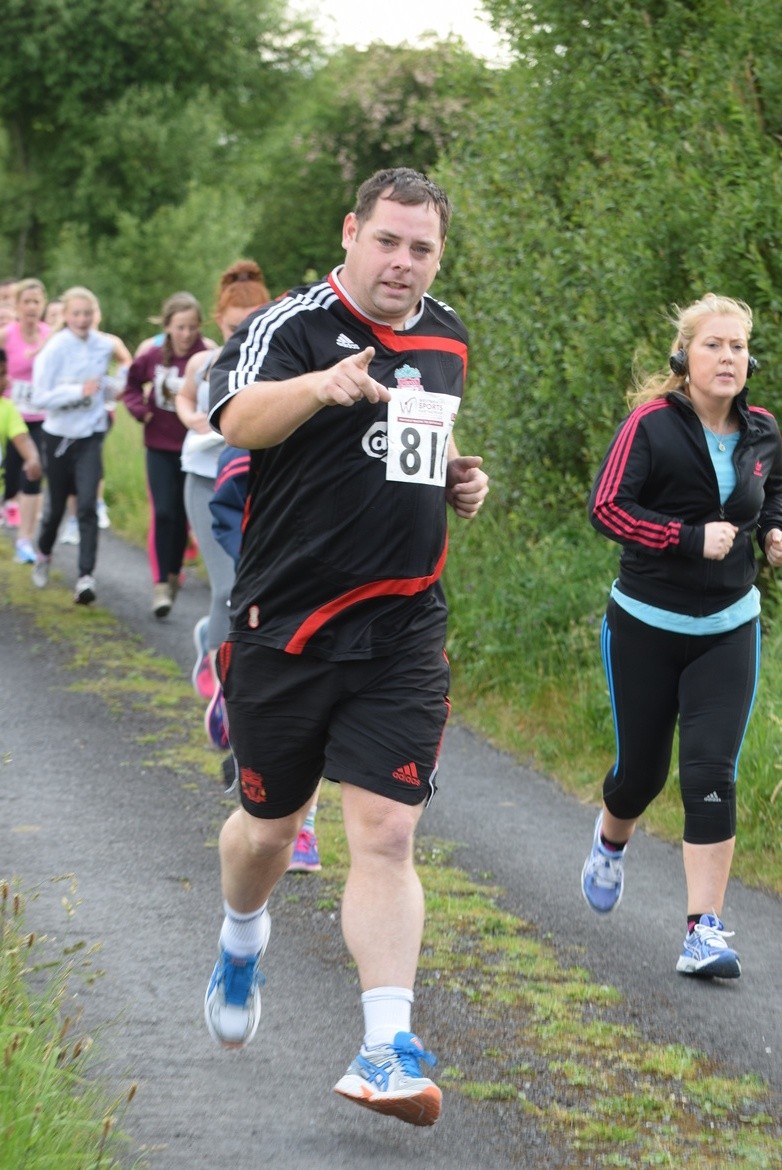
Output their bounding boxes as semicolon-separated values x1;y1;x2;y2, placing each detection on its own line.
668;347;758;379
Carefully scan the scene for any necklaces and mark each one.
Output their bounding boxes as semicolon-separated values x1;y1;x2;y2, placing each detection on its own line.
701;418;728;451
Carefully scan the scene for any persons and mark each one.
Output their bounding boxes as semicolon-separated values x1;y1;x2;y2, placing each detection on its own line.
200;168;490;1123
1;258;320;873
572;291;782;981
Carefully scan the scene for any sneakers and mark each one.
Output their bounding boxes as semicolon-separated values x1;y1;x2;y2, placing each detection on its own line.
14;539;37;565
288;829;322;872
194;616;216;699
206;687;232;749
203;909;272;1049
153;582;174;619
332;1031;443;1127
95;506;111;530
582;808;628;915
74;574;97;605
62;523;81;545
675;913;741;978
5;503;21;528
33;552;49;588
